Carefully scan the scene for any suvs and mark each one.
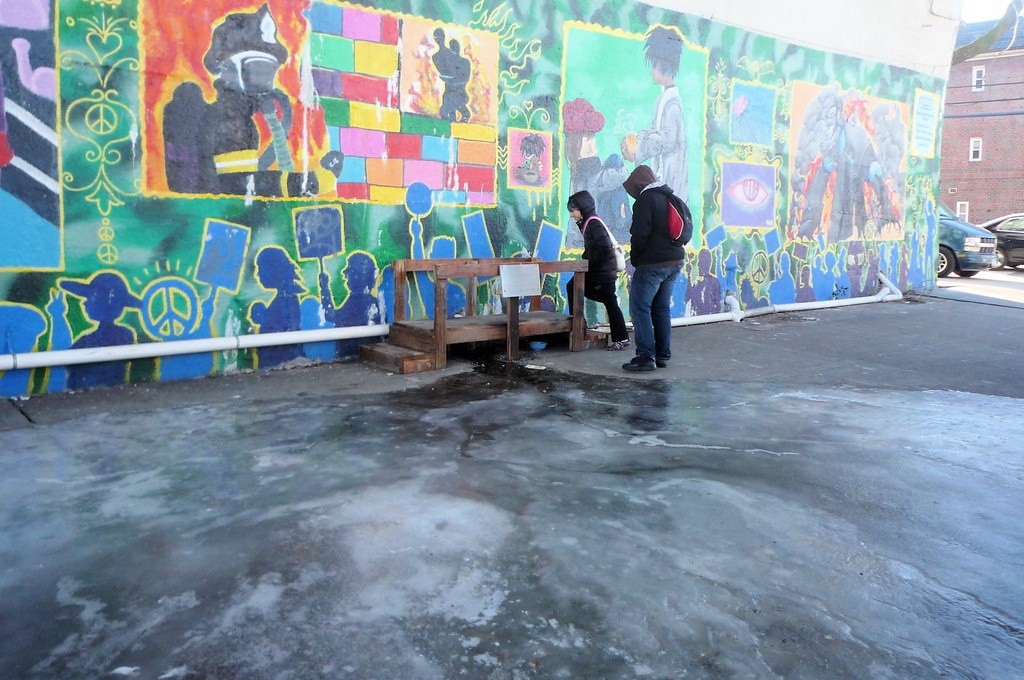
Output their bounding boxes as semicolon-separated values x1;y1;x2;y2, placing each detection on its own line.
938;198;998;278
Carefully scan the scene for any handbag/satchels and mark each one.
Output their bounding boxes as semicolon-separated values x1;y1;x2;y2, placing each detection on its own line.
582;215;626;272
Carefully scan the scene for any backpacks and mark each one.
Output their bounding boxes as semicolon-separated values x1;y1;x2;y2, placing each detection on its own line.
654;188;693;247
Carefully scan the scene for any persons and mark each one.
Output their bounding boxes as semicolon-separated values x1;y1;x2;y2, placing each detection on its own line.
566;190;630;350
623;166;685;371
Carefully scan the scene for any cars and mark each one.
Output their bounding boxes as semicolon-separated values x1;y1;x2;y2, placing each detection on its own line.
974;212;1024;271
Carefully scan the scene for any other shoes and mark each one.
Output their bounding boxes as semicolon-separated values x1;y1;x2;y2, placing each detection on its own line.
567;314;586;321
656;358;669;367
606;338;632;351
622;356;657;370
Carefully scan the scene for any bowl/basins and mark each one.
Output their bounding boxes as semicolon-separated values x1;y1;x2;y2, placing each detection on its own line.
529;342;547;351
